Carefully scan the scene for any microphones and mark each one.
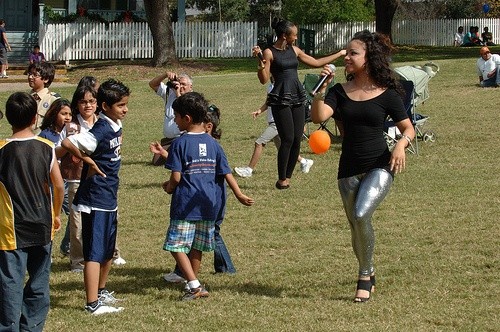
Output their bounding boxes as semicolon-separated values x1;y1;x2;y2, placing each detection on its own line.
309;63;336;97
253;45;267;64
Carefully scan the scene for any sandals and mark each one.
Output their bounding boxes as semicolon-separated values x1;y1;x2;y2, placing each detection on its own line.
353;273;375;303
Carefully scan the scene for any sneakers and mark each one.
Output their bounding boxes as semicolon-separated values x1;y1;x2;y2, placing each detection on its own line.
111;257;126;265
71;262;86;272
0;74;9;78
84;302;124;316
234;166;254;178
179;285;209;301
164;271;188;283
97;290;122;305
301;159;313;173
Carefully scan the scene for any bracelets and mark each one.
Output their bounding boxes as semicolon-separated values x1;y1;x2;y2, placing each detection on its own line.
258;64;265;70
402;135;411;148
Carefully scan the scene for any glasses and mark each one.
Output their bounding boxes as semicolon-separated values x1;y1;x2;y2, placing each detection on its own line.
79;99;97;106
27;72;41;77
482;51;489;55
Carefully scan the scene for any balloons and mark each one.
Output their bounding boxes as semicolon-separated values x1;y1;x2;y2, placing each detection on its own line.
309;130;331;154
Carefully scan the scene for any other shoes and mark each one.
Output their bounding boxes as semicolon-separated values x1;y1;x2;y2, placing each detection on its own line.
276;181;290;190
60;248;71;255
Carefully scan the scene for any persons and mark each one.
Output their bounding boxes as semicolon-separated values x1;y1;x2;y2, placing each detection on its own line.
454;26;465;47
311;30;416;303
481;27;496;46
162;92;255;303
252;16;346;190
0;91;65;332
475;47;500;87
463;26;485;47
38;98;71;256
28;45;44;65
233;73;314;178
61;79;130;316
149;104;236;282
0;20;11;78
28;61;62;135
79;75;101;91
149;71;194;165
55;85;126;272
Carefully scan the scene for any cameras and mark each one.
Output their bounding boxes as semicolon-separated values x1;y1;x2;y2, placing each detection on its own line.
166;76;176;87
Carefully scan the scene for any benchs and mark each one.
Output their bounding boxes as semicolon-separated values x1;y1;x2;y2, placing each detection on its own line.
0;60;68;83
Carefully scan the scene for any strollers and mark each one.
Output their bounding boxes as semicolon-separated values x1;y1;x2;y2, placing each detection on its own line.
385;62;440;143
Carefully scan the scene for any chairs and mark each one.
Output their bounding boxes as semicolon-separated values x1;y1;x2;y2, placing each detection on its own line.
293;73;337;140
